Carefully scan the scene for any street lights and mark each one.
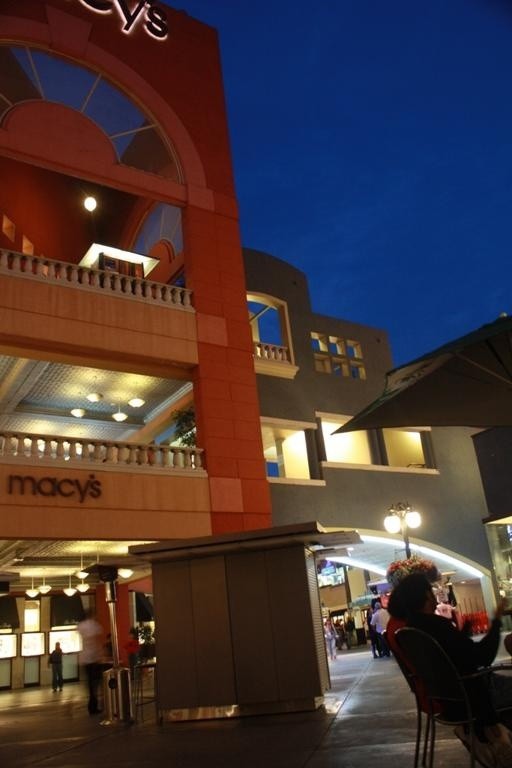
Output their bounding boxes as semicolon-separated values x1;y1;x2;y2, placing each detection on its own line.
384;500;421;560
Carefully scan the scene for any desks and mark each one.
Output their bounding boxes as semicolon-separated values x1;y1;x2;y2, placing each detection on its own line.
132;663;156;706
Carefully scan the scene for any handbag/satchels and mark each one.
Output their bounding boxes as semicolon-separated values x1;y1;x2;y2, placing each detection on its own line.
454;720;512;768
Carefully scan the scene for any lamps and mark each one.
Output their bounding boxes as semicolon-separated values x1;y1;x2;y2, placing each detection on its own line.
70;376;146;422
24;545;133;598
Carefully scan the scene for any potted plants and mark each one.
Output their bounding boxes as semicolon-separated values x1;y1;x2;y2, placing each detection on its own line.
130;617;161;660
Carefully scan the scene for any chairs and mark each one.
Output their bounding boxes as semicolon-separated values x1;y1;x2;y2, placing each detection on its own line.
382;631;486;768
394;626;512;768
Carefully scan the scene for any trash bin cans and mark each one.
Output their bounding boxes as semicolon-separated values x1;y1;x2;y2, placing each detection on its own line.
100;668;133;726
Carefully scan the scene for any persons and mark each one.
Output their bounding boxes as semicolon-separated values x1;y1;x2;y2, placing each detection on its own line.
386;572;512;734
101;630;114;658
45;640;66;694
123;628;139;681
74;605;110;719
323;600;393;659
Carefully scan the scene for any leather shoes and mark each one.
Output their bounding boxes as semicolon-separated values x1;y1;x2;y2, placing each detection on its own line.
89;709;104;715
